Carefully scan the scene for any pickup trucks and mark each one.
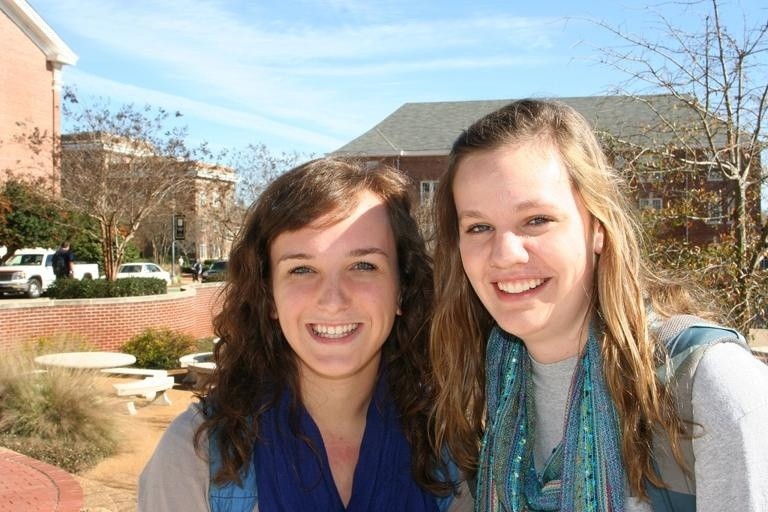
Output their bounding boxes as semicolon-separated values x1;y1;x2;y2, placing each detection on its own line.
0;247;99;298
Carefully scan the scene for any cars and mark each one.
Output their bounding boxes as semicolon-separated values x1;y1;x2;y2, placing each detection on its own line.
182;259;228;280
100;263;172;286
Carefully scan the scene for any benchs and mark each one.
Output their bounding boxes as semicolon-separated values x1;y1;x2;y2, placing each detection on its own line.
178;350;217;389
100;367;176;414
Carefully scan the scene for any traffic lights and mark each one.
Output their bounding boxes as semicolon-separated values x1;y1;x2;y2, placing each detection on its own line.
175;214;187;240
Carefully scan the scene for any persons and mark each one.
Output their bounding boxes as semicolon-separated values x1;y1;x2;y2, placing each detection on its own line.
138;156;479;512
51;240;74;281
191;258;203;281
179;255;184;273
426;97;768;512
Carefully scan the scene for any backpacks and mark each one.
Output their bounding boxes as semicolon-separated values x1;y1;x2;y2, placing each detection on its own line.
52;256;66;275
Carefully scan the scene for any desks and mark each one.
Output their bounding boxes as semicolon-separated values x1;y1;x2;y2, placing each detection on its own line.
35;352;138;370
